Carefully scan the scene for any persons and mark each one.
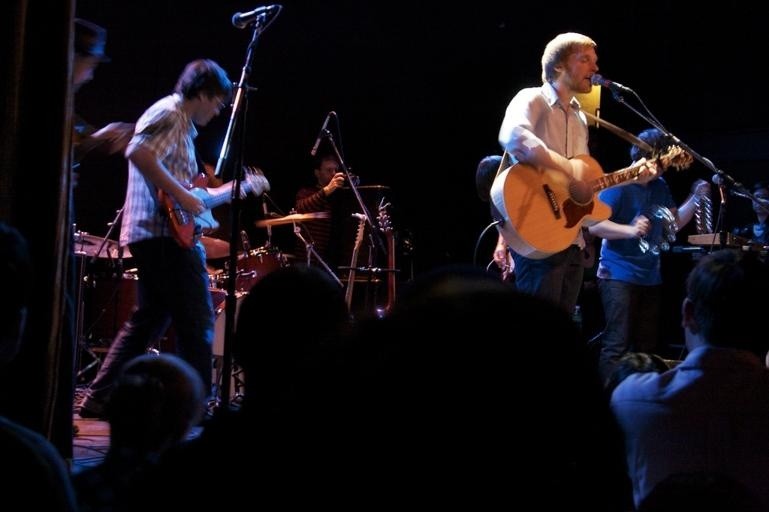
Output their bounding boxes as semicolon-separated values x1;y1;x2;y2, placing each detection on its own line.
590;128;710;386
49;17;136;435
294;157;361;267
0;223;769;512
79;59;247;427
498;32;658;320
731;181;768;245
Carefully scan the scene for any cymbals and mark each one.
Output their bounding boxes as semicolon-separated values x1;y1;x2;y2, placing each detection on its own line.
74;233;132;259
259;213;327;226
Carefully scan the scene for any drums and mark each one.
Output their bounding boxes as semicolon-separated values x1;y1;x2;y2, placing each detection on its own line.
226;247;295;293
208;287;252;401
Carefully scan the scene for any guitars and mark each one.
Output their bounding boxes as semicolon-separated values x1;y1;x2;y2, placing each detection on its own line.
159;169;272;249
490;144;693;261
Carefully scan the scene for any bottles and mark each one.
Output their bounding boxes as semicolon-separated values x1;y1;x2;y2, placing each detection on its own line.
694;190;714;235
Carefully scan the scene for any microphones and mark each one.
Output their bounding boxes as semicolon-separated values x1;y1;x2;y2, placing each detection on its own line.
232;4;277;29
711;174;736;196
590;73;633;94
310;115;331;156
